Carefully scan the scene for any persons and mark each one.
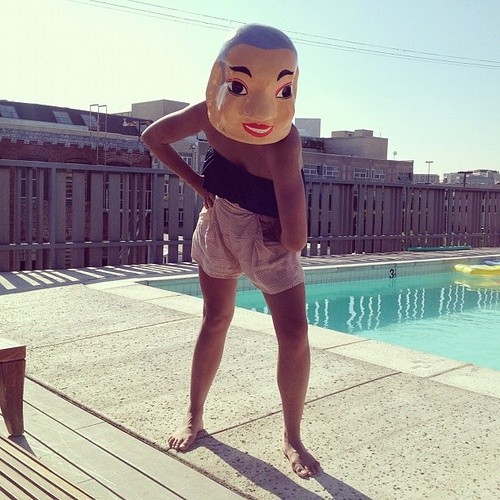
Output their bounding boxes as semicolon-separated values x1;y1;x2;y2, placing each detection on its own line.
141;24;321;477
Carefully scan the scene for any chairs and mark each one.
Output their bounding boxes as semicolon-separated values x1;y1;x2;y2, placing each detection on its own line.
0;338;26;436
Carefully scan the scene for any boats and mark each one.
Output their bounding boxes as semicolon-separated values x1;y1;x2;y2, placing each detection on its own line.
454;263;500;275
